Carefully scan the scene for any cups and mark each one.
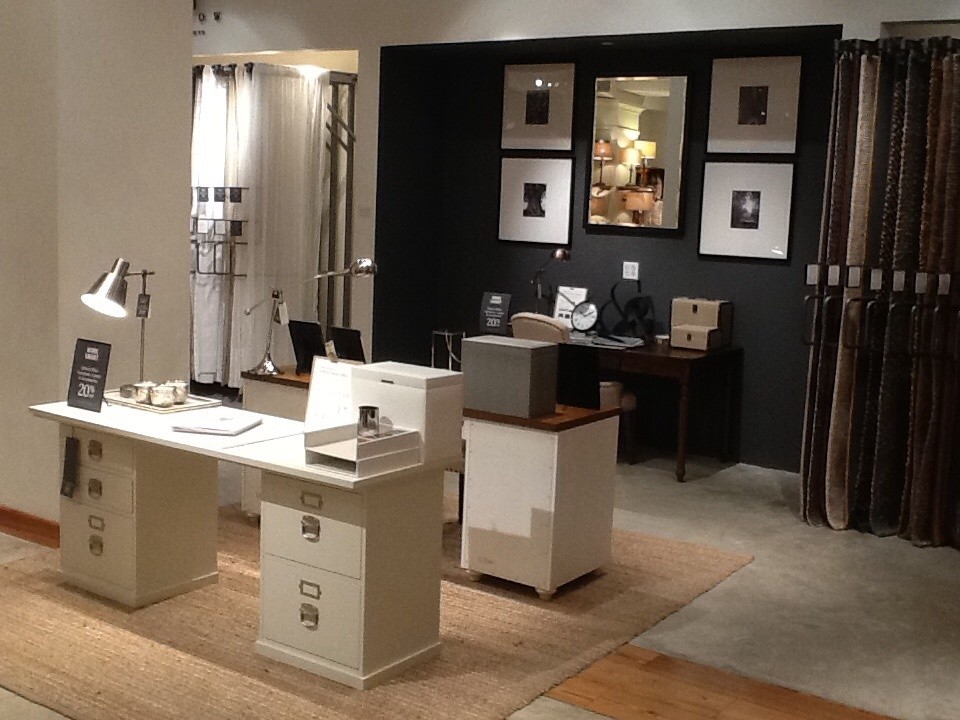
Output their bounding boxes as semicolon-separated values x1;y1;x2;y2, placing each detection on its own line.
358;406;379;438
119;378;189;407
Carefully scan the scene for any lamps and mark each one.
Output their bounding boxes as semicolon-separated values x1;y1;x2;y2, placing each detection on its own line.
80;259;155;395
592;127;656;226
533;248;571;313
244;251;380;375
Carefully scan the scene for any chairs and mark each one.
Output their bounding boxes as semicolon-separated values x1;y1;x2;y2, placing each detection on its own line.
512;312;570;344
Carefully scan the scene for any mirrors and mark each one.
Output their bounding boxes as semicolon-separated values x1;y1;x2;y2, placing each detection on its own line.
585;74;690;237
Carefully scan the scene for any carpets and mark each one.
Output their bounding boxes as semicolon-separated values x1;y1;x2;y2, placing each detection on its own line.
0;488;755;720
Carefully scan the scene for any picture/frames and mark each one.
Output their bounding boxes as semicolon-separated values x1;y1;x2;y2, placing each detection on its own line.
493;48;806;265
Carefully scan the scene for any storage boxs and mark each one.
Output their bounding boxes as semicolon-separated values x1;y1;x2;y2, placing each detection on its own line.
463;335;557;420
670;297;733;353
352;359;463;462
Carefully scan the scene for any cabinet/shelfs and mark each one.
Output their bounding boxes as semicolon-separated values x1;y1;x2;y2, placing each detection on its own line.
28;396;466;688
239;369;618;601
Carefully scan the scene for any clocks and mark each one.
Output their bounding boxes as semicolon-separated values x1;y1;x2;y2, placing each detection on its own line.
570;302;598;333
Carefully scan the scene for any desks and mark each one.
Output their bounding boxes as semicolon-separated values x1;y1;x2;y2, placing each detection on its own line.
558;337;742;481
